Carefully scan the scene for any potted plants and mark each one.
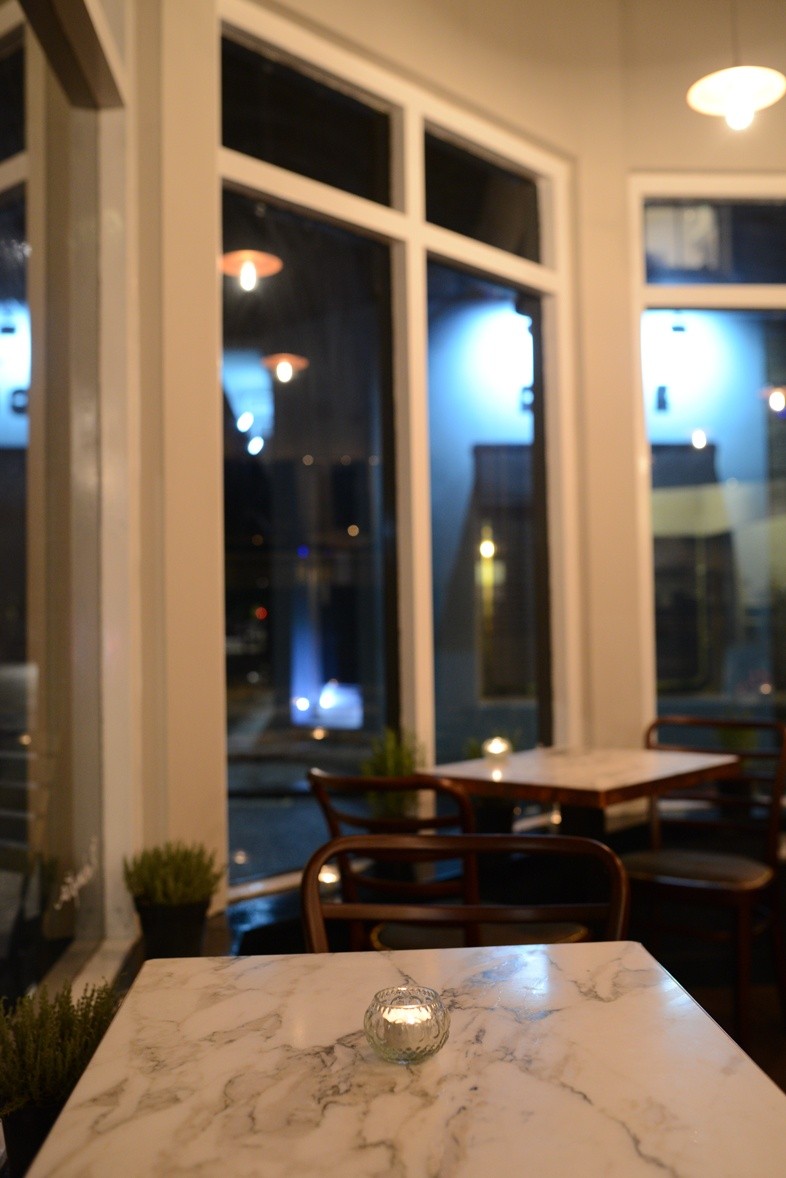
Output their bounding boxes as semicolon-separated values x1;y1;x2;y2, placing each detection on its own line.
124;847;225;962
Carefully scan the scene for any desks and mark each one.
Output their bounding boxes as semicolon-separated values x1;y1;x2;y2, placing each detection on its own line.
25;943;786;1178
413;744;743;851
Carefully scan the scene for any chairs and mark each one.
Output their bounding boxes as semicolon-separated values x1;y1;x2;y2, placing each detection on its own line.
298;718;786;942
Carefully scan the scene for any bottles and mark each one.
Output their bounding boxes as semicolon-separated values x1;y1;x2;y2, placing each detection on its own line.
363;986;453;1066
